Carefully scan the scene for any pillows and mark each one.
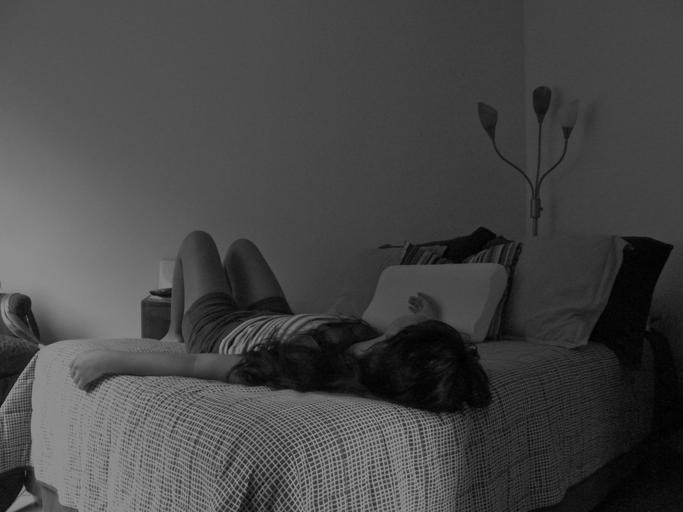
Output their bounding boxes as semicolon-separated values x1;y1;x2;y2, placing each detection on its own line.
361;264;510;346
401;240;524;340
379;228;513;264
589;234;673;359
504;231;634;349
330;246;409;324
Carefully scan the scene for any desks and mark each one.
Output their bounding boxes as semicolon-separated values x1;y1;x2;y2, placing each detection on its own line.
141;296;170;339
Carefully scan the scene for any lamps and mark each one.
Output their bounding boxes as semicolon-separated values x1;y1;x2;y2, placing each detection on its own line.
475;86;583;236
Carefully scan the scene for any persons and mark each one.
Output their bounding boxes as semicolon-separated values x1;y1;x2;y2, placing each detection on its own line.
68;229;494;415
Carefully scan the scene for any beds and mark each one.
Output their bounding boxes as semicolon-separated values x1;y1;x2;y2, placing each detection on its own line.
0;225;682;510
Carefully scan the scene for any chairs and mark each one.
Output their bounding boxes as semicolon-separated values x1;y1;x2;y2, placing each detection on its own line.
0;291;40;407
